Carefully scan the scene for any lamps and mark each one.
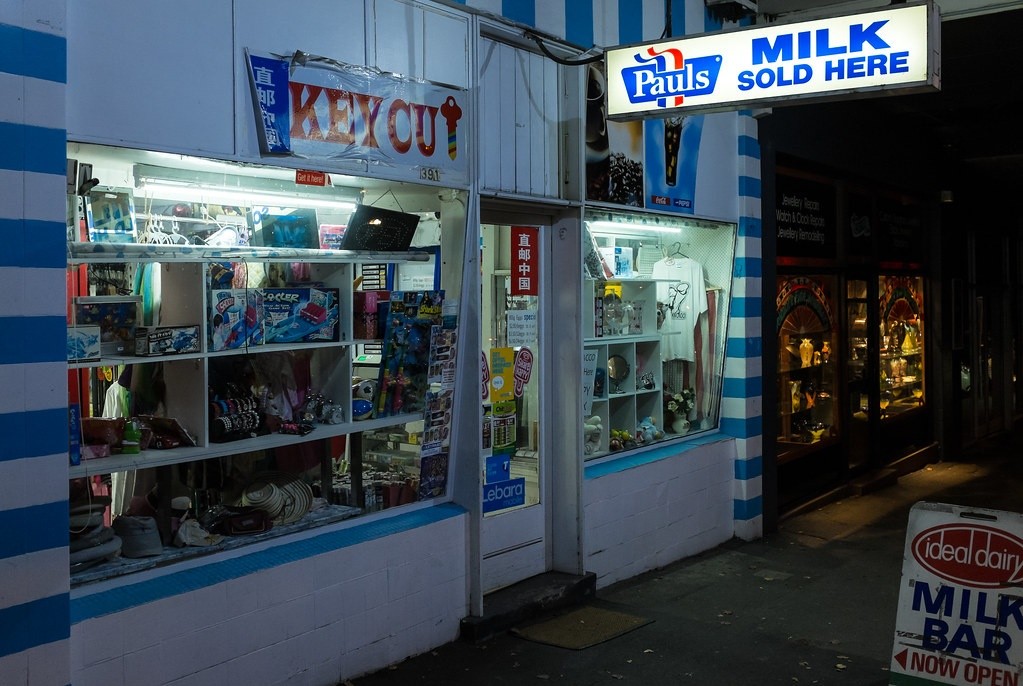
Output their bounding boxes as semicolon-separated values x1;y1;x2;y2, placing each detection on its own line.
134;164;365;211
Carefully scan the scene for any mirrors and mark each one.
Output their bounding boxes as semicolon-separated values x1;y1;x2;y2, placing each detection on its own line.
609;355;631;394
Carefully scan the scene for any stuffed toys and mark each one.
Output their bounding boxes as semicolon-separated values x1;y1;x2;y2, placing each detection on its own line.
584;416;603;453
637;417;661;443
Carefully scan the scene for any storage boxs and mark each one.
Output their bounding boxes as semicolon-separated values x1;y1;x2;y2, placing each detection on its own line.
134;325;199;358
264;287;339;344
69;324;101;363
358;313;385;339
353;290;389;314
206;288;265;353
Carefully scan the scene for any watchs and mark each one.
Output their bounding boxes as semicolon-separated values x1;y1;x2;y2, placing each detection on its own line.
212;397;257;415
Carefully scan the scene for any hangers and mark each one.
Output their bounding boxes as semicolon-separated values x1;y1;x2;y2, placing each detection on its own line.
668;242;689;259
137;212;192;245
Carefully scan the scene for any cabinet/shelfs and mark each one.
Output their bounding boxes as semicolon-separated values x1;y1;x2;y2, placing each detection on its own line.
776;265;933;506
583;278;681;459
67;255;427;589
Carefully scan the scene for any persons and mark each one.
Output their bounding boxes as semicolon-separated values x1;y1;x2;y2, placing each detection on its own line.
420;291;441;306
214;313;223;351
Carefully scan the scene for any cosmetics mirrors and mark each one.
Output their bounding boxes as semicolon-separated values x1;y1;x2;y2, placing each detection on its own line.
608;354;630;394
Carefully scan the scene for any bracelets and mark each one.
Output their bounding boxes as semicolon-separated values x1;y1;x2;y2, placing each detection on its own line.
215;410;259;433
